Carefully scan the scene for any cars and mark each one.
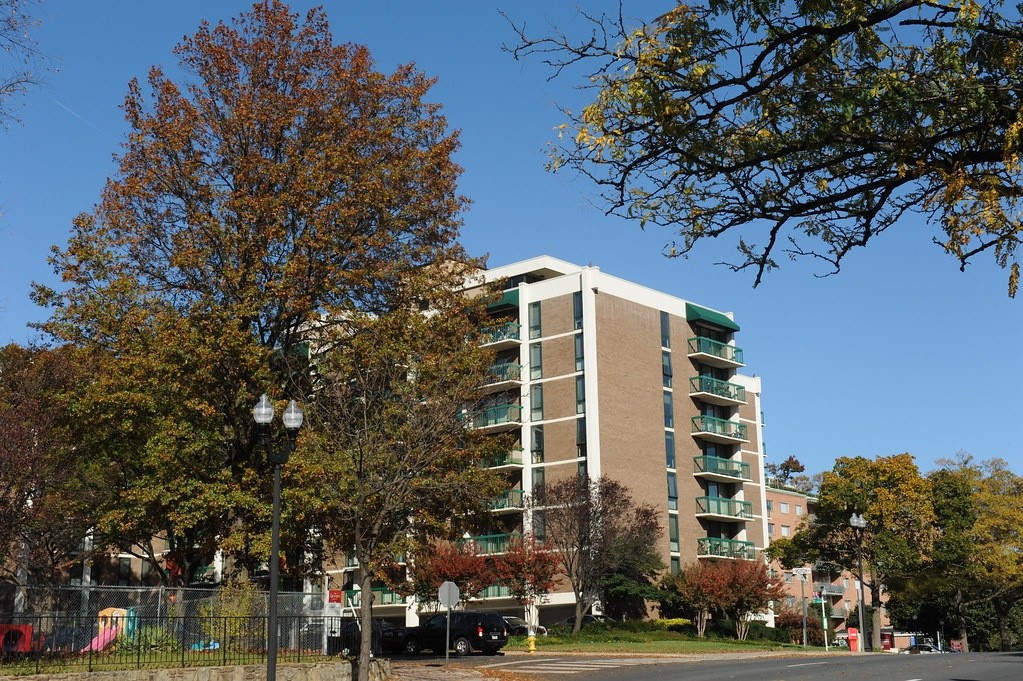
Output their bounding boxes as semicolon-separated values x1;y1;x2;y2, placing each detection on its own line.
502;616;547;637
328;621;406;657
554;614;619;627
900;644;959;655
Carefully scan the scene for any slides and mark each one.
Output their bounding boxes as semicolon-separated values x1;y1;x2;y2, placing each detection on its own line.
80;625;120;653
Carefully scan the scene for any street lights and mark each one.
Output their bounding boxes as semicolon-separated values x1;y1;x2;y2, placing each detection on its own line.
849;512;867;652
253;393;304;681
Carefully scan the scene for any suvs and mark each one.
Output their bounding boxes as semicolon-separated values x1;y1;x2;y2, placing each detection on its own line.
402;611;508;657
